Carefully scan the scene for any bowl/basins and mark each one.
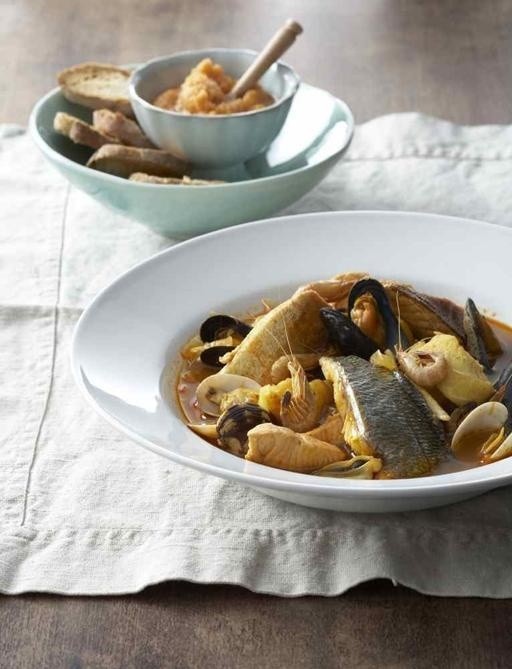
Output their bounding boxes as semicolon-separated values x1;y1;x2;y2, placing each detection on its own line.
27;73;354;240
126;48;302;168
70;209;509;520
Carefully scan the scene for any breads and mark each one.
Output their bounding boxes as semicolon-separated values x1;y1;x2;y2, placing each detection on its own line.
48;63;229;185
